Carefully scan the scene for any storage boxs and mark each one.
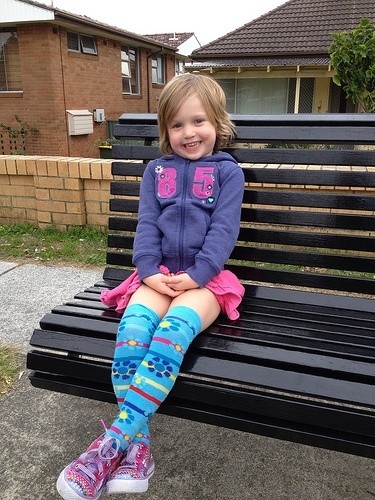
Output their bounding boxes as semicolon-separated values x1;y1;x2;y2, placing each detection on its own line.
66;109;94;136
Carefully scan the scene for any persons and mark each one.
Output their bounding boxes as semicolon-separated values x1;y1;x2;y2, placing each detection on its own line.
56;72;245;500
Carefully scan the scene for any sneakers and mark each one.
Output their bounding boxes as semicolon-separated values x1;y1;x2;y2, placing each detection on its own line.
106;442;155;494
56;418;125;500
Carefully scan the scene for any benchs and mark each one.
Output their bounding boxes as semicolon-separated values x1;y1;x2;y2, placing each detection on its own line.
25;112;375;460
